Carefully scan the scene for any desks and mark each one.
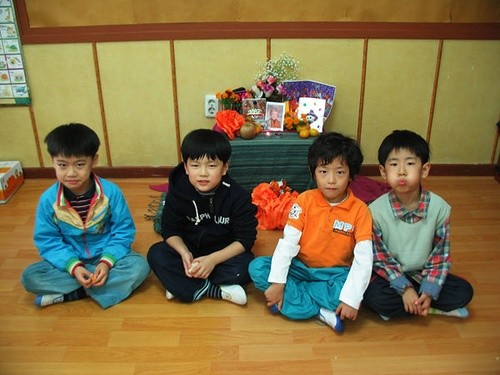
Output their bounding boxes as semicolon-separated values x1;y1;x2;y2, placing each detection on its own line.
227;132;316;194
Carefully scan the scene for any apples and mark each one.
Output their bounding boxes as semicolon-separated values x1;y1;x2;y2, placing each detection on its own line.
239;123;256;139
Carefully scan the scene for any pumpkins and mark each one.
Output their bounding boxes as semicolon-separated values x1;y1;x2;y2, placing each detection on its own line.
296;121;309;132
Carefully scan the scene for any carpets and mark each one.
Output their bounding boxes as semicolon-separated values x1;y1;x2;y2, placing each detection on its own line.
150;175;391;204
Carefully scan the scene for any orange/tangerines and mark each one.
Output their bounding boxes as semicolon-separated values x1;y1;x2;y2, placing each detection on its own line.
300;128;318;138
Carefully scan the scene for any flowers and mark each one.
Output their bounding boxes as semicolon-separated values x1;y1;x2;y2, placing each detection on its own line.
216;50;304;114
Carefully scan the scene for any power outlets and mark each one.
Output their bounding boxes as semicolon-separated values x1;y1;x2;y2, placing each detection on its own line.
204;95;218;118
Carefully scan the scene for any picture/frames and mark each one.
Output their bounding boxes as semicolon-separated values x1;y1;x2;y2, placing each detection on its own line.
265;102;285;132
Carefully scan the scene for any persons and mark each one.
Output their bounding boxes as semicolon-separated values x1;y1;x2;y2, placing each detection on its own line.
146;129;258;305
268;106;281;128
20;123;151;310
360;129;473;320
248;132;373;333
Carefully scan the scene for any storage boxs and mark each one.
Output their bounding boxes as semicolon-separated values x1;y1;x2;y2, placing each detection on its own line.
0;161;24;204
